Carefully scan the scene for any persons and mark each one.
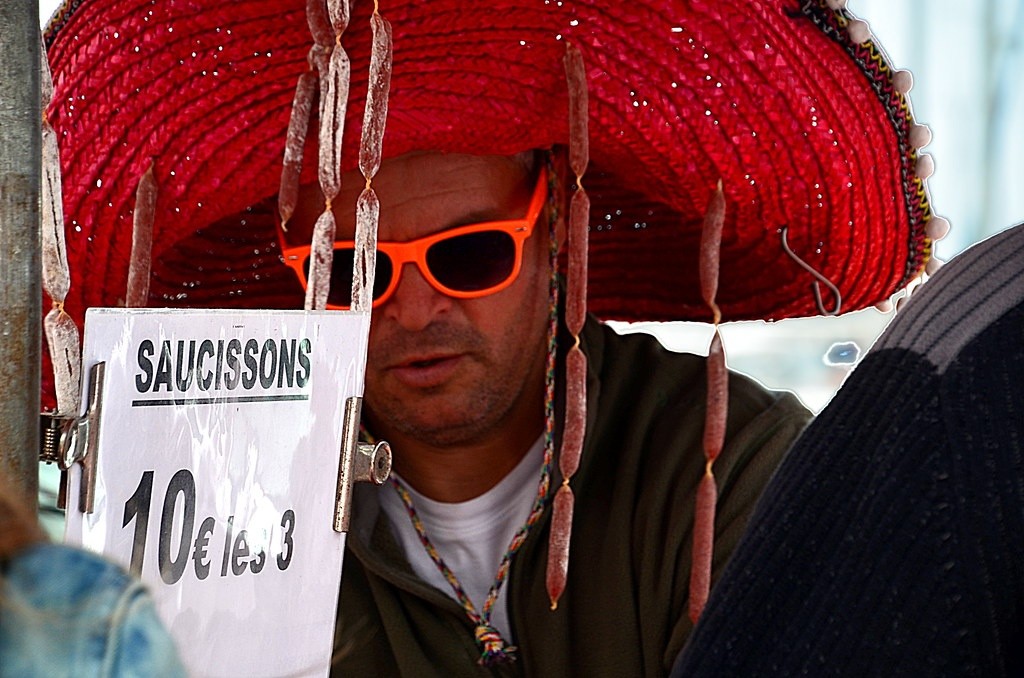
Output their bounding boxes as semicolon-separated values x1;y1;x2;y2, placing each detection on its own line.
1;0;1023;678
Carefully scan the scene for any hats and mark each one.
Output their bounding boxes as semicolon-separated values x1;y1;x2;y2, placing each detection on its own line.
43;0;948;412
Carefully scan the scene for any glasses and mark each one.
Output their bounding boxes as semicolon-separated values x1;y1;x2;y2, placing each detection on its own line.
273;155;550;310
